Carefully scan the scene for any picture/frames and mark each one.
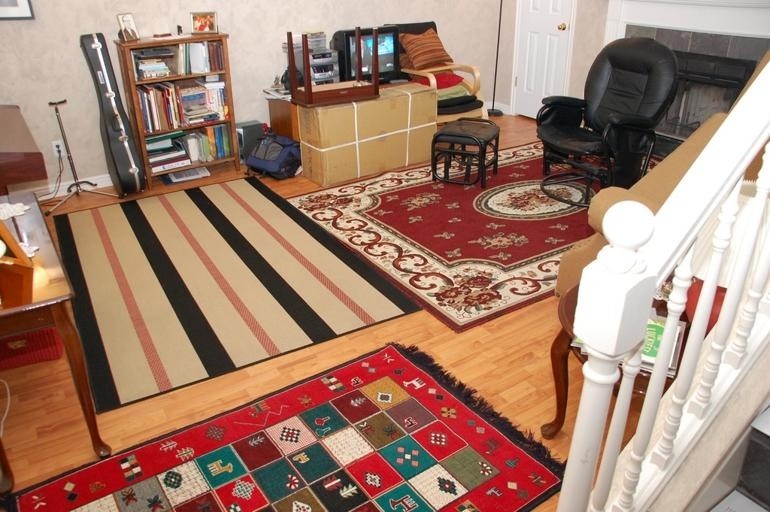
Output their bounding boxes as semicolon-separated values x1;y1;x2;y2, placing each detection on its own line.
188;9;220;35
0;0;35;21
115;12;140;43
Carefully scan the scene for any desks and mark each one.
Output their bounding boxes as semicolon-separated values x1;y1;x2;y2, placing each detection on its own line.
541;280;690;445
0;189;113;460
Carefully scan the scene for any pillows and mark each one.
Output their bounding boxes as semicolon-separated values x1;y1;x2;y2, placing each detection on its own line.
396;26;472;104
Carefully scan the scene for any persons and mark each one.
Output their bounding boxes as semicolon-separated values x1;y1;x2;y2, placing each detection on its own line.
353;39;372;76
122;15;137;42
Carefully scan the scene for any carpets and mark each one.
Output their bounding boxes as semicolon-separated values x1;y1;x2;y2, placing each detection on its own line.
1;340;567;511
51;173;426;416
1;326;64;375
282;138;669;335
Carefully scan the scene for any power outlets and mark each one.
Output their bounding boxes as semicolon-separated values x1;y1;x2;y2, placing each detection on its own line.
53;139;68;160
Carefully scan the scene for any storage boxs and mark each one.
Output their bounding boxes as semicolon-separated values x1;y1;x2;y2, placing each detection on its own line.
299;84;439;188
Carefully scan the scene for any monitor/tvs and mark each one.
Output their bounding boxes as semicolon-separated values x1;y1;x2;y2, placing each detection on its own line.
329;28;398;85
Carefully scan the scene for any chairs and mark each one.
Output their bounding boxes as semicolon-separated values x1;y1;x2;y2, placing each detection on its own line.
535;35;680;208
385;20;492;153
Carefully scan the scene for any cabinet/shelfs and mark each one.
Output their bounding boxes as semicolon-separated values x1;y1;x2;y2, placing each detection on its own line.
112;33;242;193
269;99;298;142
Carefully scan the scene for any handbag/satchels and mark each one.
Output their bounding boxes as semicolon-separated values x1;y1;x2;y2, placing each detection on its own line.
244;128;300;181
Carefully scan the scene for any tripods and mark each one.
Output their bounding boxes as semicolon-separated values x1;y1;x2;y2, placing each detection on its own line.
45;99;127;216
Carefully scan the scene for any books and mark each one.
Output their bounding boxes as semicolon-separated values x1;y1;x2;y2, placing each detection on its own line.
570;307;689;382
131;40;235;183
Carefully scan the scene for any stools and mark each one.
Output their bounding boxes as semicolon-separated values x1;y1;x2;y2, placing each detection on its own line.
431;117;502;190
283;25;380;108
